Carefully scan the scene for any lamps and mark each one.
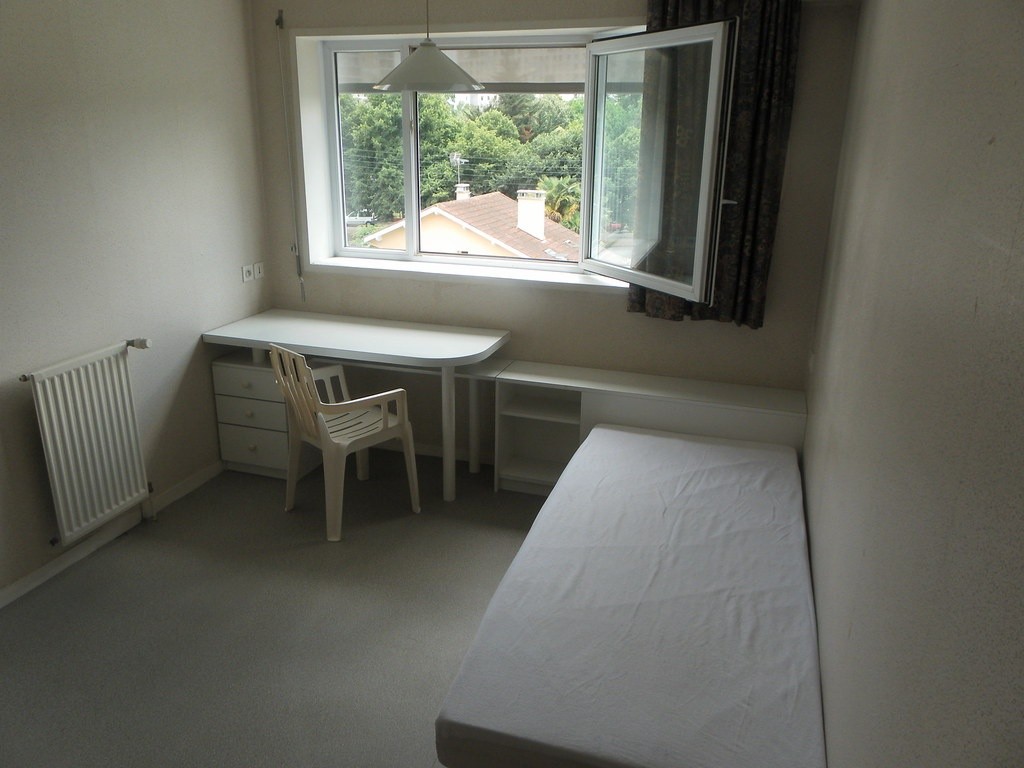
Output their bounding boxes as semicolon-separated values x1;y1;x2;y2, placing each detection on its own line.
372;0;486;92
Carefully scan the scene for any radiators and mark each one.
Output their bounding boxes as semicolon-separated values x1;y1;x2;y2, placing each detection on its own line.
18;337;152;549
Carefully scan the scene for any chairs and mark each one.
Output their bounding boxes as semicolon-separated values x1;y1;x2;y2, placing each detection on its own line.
269;343;421;541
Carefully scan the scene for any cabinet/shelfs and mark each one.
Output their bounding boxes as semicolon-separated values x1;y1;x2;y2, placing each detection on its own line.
209;352;513;513
493;360;807;495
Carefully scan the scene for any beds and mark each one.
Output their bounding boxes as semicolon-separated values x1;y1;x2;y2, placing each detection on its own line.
435;422;826;768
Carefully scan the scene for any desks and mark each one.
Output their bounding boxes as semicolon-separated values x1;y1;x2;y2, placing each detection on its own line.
202;307;511;501
